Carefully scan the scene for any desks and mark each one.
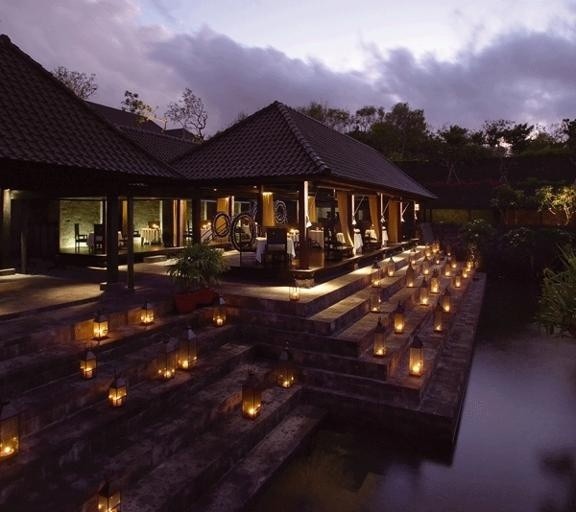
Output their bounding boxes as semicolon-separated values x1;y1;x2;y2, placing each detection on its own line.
72;220;389;262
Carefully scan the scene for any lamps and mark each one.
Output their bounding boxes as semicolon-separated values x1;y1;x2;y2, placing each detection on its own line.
360;238;481;379
